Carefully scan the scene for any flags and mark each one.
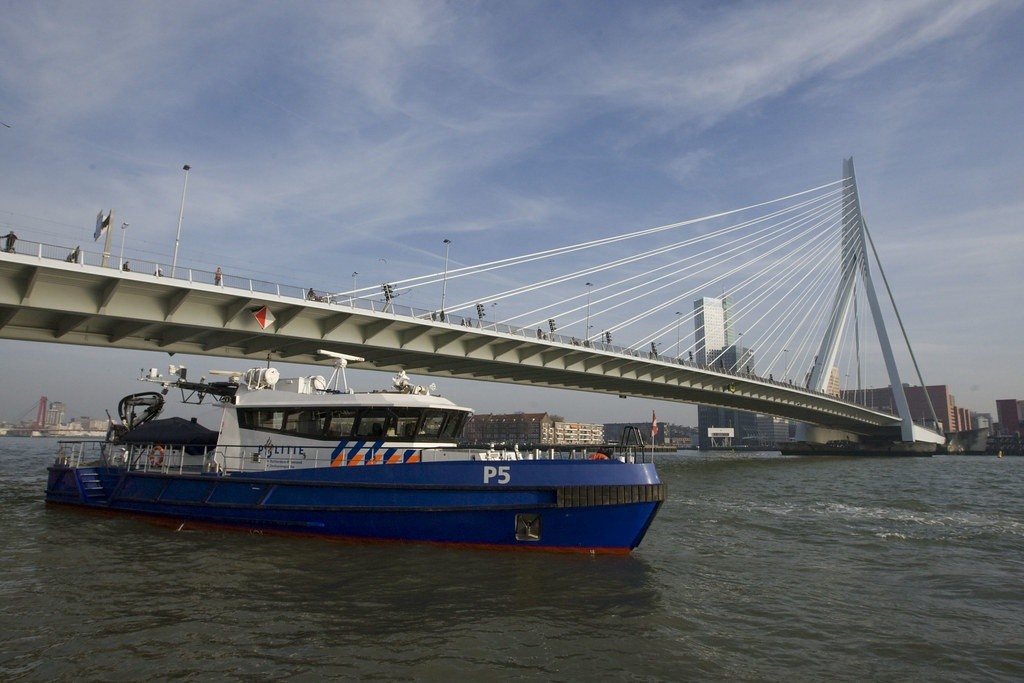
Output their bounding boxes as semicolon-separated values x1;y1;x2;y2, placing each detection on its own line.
651;411;659;438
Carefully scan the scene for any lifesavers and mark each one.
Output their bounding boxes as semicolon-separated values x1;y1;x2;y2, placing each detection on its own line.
150;446;163;464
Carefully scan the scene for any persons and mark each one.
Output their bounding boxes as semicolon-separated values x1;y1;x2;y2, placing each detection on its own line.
213;265;222;285
598;446;613;459
118;447;128;466
537;326;544;340
306;288;316;300
440;310;445;322
0;230;18;253
154;267;164;277
122;261;131;272
432;311;437;321
75;245;81;263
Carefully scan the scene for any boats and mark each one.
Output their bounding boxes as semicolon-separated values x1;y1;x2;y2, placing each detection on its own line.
42;346;669;561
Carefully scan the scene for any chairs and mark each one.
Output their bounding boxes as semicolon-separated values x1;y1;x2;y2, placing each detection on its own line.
368;422;419;437
